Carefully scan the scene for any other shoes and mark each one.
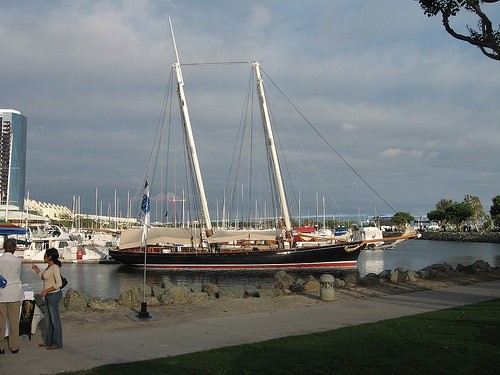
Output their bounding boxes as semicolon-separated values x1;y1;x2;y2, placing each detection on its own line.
0;349;5;354
12;347;19;354
47;344;63;351
39;343;48;347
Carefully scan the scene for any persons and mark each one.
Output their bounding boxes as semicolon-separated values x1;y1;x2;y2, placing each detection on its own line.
32;247;63;350
0;238;23;354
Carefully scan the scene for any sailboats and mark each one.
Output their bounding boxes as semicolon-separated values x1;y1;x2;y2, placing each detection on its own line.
110;17;421;272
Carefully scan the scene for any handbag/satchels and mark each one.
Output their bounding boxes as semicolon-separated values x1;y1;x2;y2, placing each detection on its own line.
53;273;68;289
0;275;7;288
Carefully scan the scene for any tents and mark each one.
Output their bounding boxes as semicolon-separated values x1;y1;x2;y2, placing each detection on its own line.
0;219;26;248
207;230;284;243
120;227;201;250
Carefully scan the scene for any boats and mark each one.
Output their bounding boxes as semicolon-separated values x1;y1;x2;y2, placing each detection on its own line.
21;187;139;263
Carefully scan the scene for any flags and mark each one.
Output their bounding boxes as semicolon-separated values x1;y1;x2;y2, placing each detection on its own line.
139;179;151;244
